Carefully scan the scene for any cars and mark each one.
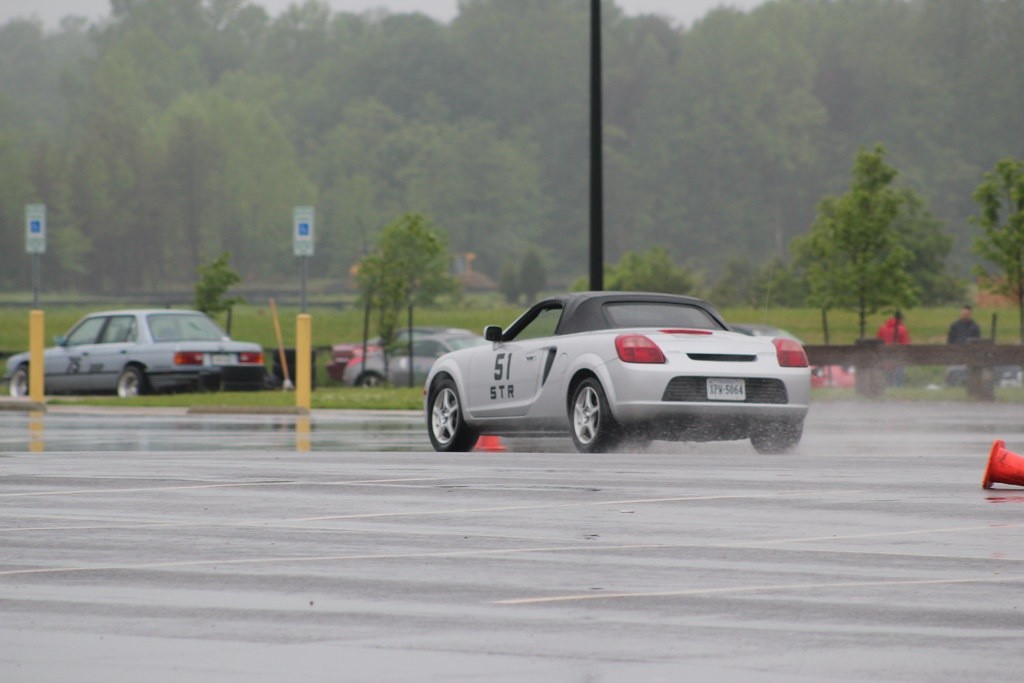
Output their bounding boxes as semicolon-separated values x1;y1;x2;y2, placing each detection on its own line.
325;326;429;382
809;362;857;390
341;331;487;390
5;307;267;402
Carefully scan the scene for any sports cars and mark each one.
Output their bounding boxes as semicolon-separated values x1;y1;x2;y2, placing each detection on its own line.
423;289;810;454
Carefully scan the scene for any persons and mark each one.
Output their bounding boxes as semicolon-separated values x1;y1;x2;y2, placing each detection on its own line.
876;308;910;383
946;304;979;389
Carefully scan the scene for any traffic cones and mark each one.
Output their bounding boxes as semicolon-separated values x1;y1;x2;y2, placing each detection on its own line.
474;435;507;451
982;439;1024;489
